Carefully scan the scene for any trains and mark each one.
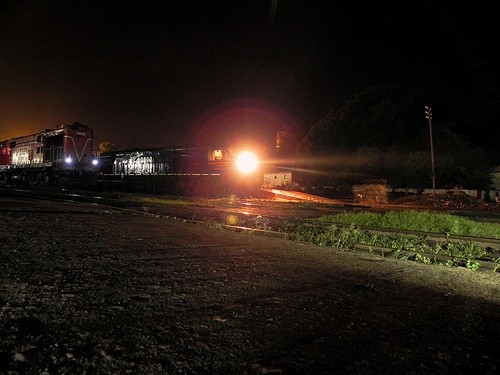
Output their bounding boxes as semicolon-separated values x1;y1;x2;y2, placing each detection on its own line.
93;141;268;194
0;123;96;189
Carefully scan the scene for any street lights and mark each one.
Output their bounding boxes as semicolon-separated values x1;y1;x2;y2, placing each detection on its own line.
425;106;436;202
